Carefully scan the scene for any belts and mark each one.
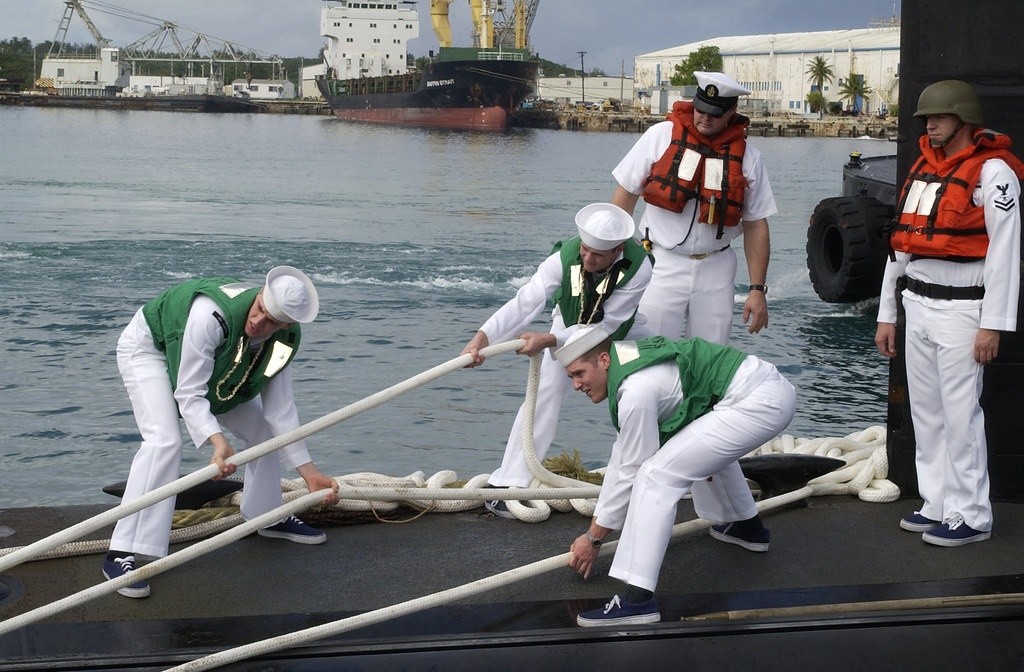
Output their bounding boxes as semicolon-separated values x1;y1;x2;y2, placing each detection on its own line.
689;244;730;259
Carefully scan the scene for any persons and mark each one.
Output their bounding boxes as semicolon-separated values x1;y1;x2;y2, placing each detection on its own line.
554;324;796;627
875;80;1024;546
101;266;340;597
461;205;654;518
612;71;778;346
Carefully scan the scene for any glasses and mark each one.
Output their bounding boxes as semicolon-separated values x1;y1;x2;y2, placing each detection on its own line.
695;107;724;118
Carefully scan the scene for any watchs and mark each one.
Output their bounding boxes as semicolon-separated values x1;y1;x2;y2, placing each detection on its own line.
585;530;604;548
749;284;769;295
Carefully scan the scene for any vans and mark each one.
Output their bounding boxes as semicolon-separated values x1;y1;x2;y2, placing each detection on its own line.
593;99;605;109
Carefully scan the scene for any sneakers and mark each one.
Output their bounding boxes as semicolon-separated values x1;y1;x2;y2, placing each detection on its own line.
710;525;770;551
102;552;151;598
485;500;516;519
922;520;991;546
577;595;661;627
258;514;327;544
900;513;940;532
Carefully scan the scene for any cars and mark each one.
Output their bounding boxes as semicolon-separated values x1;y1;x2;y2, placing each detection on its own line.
235;91;251;99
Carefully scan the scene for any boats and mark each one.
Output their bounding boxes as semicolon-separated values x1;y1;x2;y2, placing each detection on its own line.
315;47;540;133
807;152;897;304
0;78;48;106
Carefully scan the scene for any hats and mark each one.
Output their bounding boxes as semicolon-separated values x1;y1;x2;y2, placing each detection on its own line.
693;71;751;115
574;203;635;251
263;266;319;324
913;80;984;126
554;324;609;368
